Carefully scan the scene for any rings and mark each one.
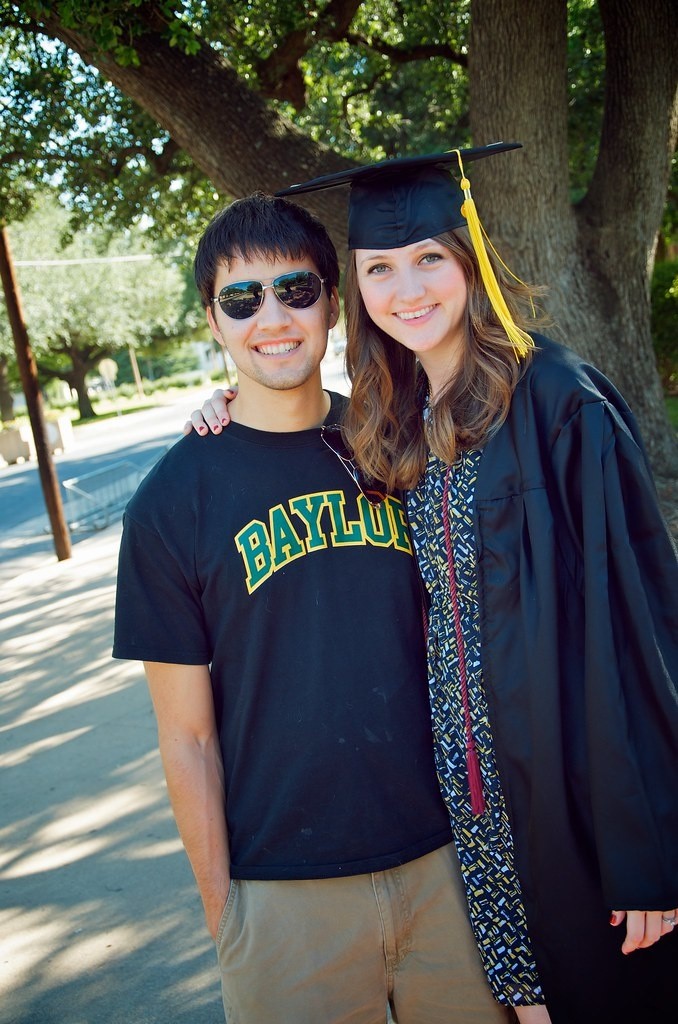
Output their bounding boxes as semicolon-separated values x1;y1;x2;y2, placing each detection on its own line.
663;917;677;925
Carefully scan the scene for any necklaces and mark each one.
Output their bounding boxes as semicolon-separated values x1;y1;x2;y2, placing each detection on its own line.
427;381;434;405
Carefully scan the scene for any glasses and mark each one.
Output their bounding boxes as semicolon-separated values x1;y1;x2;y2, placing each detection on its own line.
319;423;389;509
210;269;335;321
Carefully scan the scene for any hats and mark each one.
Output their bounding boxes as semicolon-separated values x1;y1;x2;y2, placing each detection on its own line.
273;144;535;364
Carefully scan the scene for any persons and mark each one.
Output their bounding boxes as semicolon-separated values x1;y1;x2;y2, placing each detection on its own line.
184;140;678;1024
108;190;513;1024
